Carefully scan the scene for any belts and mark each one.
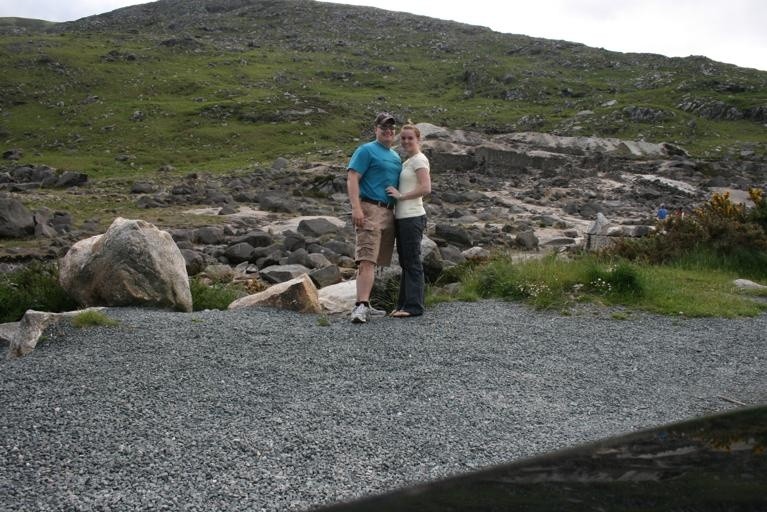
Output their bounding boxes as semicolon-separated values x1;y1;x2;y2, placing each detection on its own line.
361;196;395;209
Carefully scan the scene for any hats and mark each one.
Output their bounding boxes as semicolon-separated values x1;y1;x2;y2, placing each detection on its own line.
375;112;397;124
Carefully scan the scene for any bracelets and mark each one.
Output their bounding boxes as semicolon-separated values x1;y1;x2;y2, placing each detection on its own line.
399;192;401;199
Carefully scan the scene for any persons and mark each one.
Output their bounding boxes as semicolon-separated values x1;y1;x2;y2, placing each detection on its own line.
346;112;403;324
654;203;672;219
384;124;431;319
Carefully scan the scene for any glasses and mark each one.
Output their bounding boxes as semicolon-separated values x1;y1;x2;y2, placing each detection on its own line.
376;124;397;130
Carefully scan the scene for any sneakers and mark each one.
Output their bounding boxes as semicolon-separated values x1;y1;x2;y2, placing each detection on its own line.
366;303;387;318
389;308;423;319
350;303;370;323
